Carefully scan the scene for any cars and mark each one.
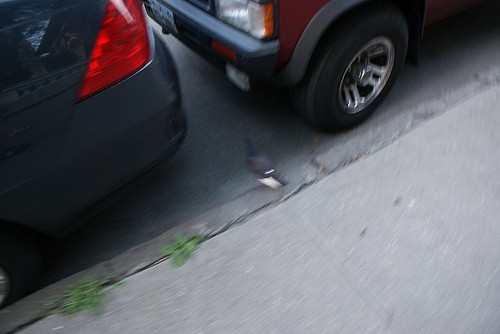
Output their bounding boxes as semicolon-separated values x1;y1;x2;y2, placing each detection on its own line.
144;0;479;133
0;0;189;310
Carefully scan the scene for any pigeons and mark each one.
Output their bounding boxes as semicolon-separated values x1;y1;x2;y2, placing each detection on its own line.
240;135;288;187
